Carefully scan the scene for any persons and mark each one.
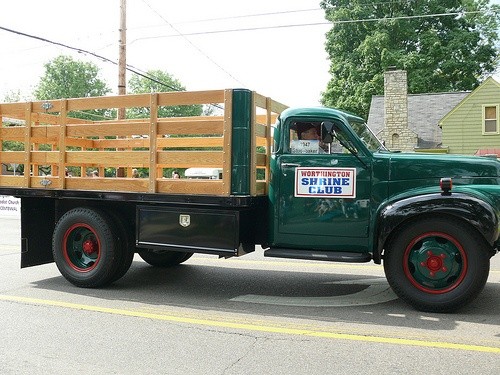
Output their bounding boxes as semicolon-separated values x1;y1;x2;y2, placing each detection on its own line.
296;123;327;154
4;164;182;182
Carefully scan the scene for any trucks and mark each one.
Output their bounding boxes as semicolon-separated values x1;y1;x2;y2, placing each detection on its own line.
0;89;500;315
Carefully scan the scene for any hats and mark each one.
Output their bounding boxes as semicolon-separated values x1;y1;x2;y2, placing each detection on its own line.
296;122;314;134
